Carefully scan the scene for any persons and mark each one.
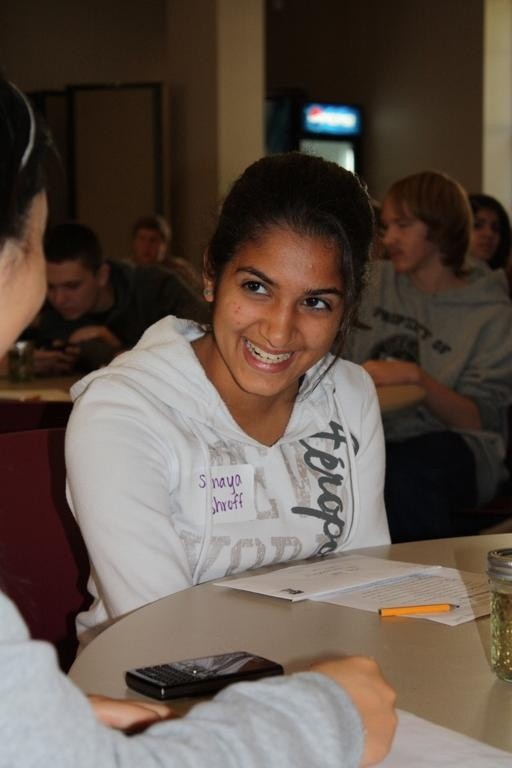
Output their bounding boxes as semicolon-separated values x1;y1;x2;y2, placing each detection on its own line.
61;146;395;665
23;168;511;543
2;79;399;765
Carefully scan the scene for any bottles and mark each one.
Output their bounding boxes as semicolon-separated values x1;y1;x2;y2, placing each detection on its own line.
482;545;512;687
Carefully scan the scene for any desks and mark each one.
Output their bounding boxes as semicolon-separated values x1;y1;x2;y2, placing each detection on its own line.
55;525;512;768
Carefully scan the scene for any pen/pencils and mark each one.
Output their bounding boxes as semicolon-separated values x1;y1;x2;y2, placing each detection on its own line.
378;602;461;618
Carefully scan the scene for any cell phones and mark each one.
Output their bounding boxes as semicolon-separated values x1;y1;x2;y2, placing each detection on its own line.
125;650;285;700
